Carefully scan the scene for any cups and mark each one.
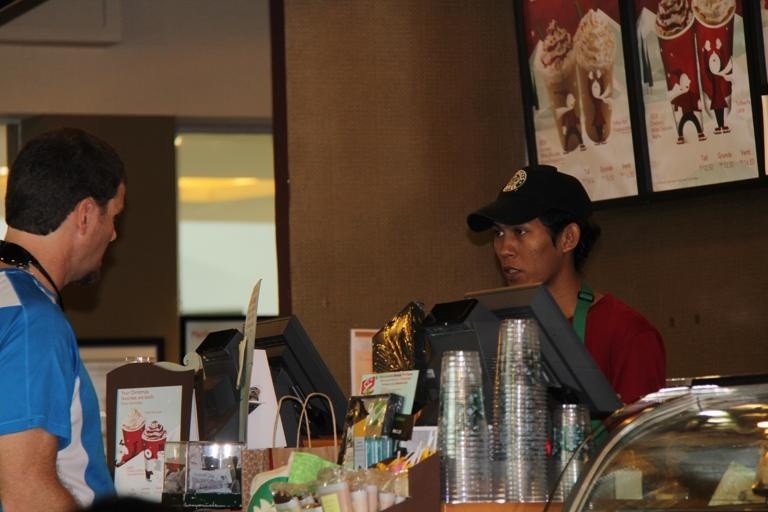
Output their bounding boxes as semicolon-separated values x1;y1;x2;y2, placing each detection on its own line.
655;8;735;145
535;39;617;152
120;426;167;484
441;318;596;504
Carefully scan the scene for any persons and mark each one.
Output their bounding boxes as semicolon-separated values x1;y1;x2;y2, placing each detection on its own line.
671;39;733;144
555;69;613;154
0;128;127;512
467;165;667;466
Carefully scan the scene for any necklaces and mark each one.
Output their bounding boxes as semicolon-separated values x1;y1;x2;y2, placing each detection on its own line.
0;240;64;312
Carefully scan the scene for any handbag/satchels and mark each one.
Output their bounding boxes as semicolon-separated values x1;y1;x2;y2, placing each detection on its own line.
262;392;344;475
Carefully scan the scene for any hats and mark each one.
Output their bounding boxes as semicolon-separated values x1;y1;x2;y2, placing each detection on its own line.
467;164;594;233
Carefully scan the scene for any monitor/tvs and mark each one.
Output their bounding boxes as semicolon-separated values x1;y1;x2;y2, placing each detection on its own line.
244;315;348;436
464;282;623;413
